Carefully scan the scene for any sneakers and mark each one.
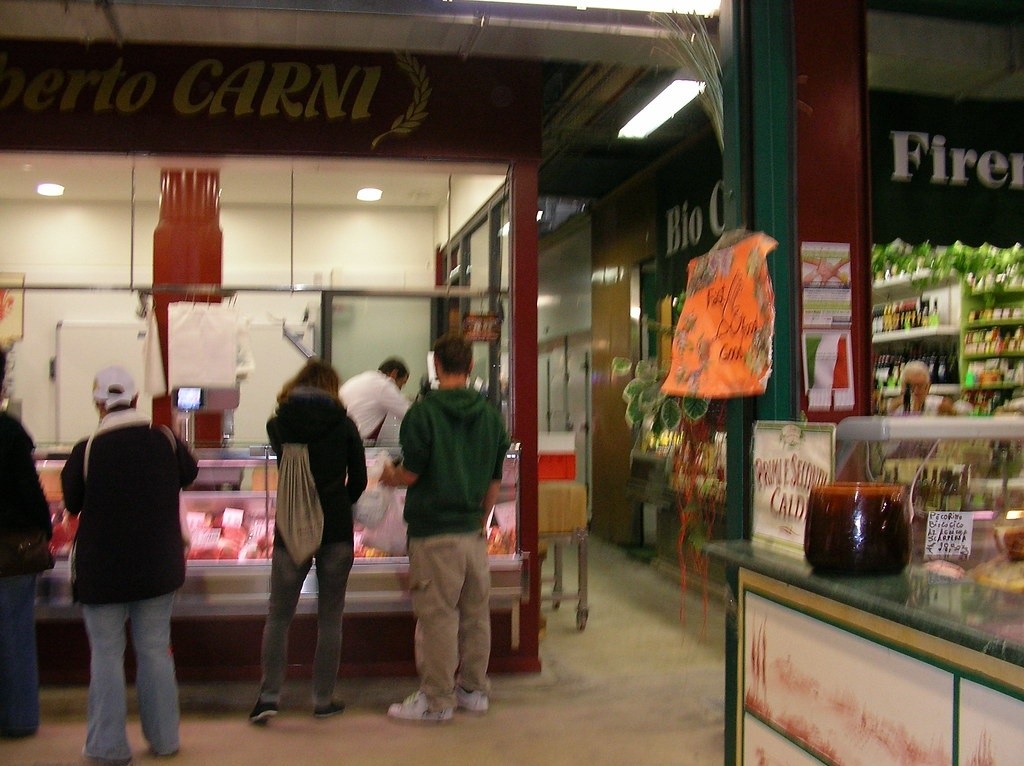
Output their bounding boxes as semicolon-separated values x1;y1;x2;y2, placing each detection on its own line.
386;686;491;721
248;699;278;722
312;700;346;717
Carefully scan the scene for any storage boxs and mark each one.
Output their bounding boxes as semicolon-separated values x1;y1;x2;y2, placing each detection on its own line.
536;426;587;533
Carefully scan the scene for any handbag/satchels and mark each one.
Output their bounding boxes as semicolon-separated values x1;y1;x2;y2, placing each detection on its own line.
275;445;325;564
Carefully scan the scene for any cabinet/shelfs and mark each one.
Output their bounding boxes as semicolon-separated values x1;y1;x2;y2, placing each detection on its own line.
872;267;960;417
959;272;1024;417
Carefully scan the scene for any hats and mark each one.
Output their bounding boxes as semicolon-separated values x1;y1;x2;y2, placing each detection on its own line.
93;366;139;408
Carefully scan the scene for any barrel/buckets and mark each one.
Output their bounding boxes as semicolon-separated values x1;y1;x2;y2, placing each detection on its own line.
805;482;912;577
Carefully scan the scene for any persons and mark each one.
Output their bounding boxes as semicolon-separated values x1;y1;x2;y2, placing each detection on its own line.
380;335;511;722
247;360;367;723
887;362;953;417
61;365;199;766
338;357;413;446
0;351;56;740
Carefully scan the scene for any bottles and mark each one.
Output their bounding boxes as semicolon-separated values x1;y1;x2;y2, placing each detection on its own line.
874;355;894;416
872;300;938;334
894;356;905;385
964;390;1000;416
918;351;958;384
884;462;970;513
904;382;912;416
906;356;916;365
